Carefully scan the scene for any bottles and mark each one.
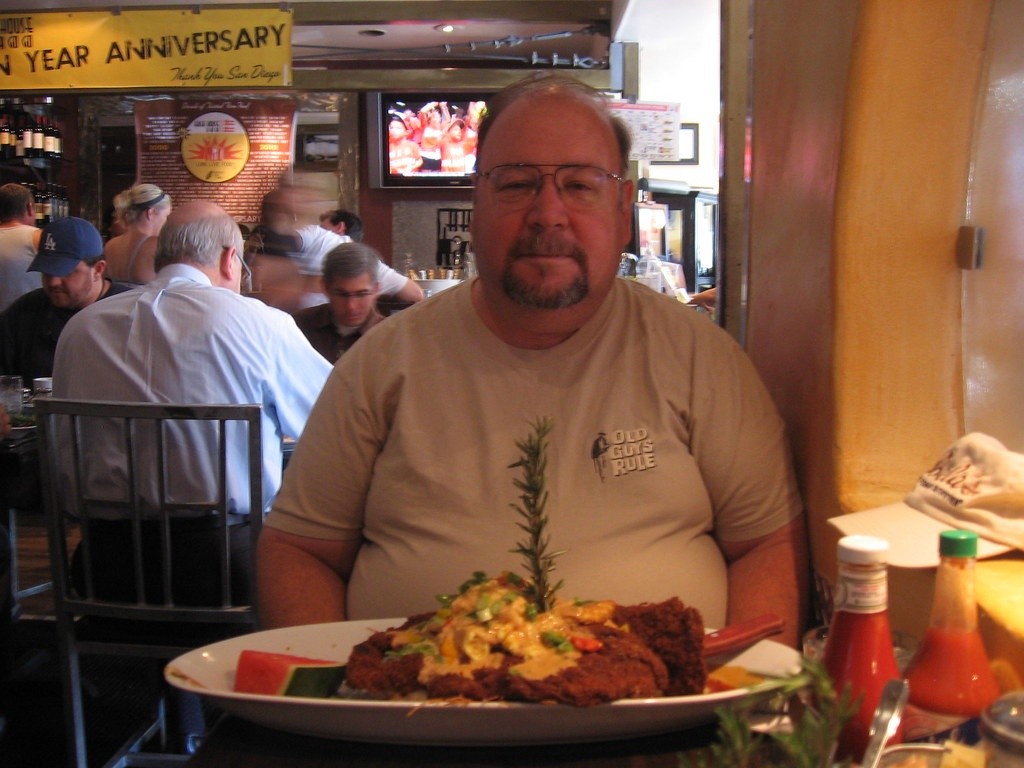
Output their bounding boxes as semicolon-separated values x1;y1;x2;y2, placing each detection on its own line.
0;104;69;229
806;534;904;768
901;530;999;748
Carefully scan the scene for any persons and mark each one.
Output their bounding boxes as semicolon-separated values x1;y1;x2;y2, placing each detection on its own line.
0;215;155;391
229;187;330;310
386;100;487;174
279;208;423;315
290;243;386;364
0;403;12;441
101;184;172;293
0;183;46;311
254;75;805;650
51;199;336;750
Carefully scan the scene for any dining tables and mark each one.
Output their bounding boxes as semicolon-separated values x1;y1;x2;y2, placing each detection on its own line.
170;698;872;768
1;406;50;617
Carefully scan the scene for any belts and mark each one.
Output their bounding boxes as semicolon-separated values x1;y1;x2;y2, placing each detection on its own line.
86;513;248;536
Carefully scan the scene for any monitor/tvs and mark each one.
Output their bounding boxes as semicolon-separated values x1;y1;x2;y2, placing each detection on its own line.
366;88;497;189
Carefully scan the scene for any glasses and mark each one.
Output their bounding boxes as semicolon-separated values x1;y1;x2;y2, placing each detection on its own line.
475;164;624;211
222;245;251;286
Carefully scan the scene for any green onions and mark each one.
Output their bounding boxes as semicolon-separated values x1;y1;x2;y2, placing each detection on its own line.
383;571;598;659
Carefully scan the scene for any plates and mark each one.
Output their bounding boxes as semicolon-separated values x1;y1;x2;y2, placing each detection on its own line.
162;614;806;747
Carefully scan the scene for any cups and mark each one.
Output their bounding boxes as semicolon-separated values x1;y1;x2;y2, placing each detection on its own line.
0;375;23;418
978;689;1024;768
33;377;52;397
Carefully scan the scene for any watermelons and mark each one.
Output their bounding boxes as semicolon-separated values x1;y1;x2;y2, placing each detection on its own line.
234;649;347;701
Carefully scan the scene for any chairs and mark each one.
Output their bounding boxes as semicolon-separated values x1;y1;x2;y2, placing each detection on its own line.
30;396;266;768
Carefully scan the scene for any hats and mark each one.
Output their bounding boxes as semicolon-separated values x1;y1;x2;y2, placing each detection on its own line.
27;216;104;276
827;433;1024;568
